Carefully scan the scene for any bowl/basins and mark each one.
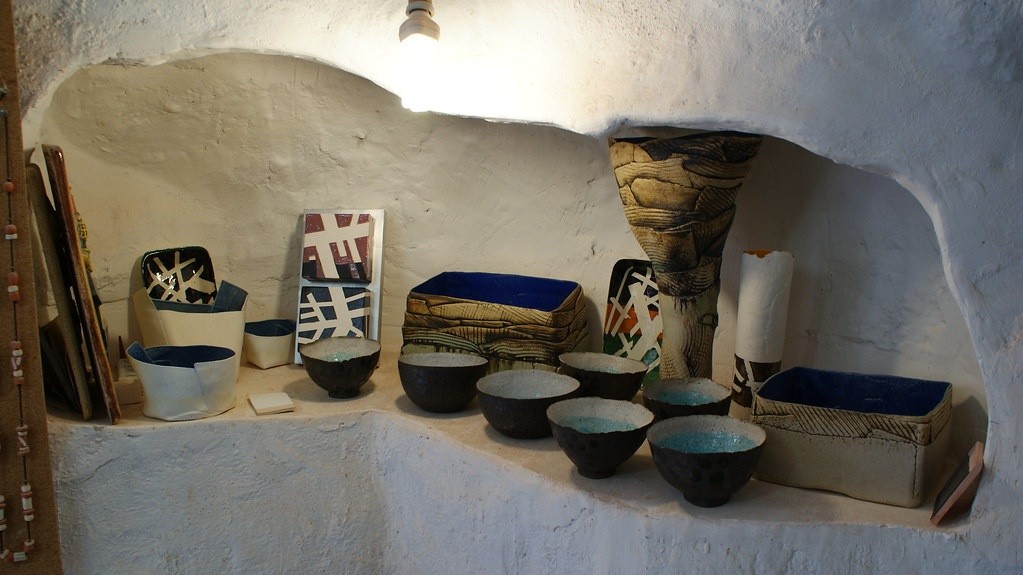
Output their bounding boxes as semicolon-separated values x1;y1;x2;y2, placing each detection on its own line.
642;377;733;420
476;369;580;441
398;352;488;414
647;415;767;508
558;351;649;402
299;336;382;399
546;397;655;479
